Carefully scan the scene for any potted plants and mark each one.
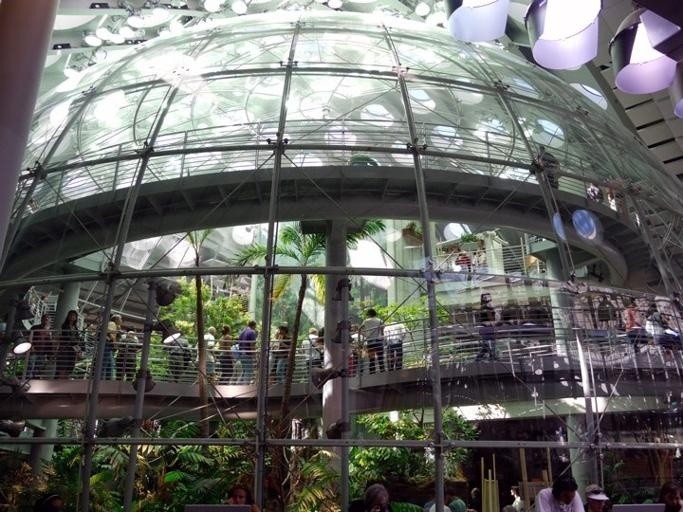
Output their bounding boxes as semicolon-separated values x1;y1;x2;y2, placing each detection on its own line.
458;234;479;251
402;222;424;244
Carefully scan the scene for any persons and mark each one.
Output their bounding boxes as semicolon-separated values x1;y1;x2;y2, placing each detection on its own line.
346;150;378;167
454;248;488;283
34;492;65;511
169;331;190;383
475;293;503;362
17;198;41;219
496;297;528;356
360;308;406;375
203;320;258;386
594;290;683;357
222;482;260;512
521;296;553;355
35;294;48;316
532;475;586;512
27;315;55;378
270;326;291;387
582;484;610;512
586;181;640;227
660;481;682;512
407;485;481;512
509;484;524;511
346;483;394;512
529;144;562;189
56;311;85;380
100;315;139;381
302;327;324;374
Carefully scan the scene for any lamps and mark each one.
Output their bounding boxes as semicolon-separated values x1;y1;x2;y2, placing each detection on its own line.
152;319;182;344
9;336;32;354
673;63;683;119
524;0;603;70
444;0;510;43
608;8;678;94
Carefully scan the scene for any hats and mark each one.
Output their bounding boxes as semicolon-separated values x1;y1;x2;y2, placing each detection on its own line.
585;484;610;501
